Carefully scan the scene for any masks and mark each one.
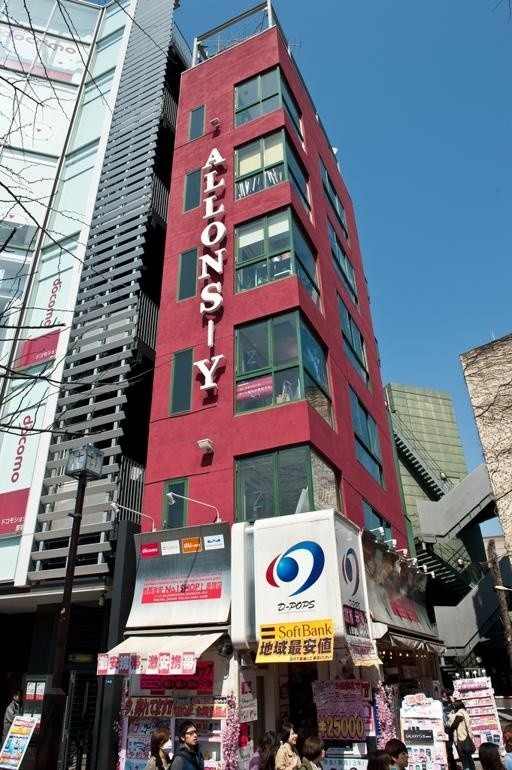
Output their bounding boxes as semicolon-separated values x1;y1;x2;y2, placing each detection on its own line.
162;739;173;753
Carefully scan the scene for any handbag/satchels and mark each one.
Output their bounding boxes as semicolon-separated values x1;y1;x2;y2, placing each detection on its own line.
456;734;476;756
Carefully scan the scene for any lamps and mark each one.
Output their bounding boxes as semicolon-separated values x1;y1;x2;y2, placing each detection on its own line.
423;571;436;579
197;439;213;454
413;565;427;573
99;592;106;606
370;526;385;544
209;118;220;128
110;502;154;532
166;492;221;524
396;548;408;558
384;539;397;552
406;558;417;566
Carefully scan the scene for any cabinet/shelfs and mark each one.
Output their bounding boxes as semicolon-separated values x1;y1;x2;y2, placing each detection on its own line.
452;677;506;759
120;717;227;770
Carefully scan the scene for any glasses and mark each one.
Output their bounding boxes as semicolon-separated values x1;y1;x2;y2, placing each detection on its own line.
185;730;199;736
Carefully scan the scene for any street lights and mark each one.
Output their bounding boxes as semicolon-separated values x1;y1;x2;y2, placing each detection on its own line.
35;444;105;770
494;585;512;592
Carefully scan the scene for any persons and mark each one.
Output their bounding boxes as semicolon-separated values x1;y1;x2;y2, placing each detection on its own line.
477;741;508;770
444;699;477;770
366;750;392;770
274;719;301;770
169;718;205;770
296;734;327;770
501;723;512;770
439;688;457;770
0;689;20;749
249;730;281;769
143;727;173;770
385;739;410;770
